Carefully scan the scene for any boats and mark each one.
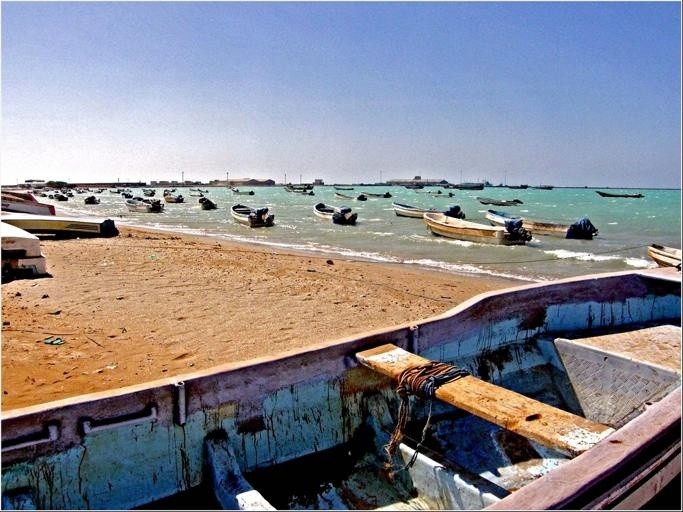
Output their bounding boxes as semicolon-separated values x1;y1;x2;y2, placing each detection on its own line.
648;244;683;266
2;264;682;511
